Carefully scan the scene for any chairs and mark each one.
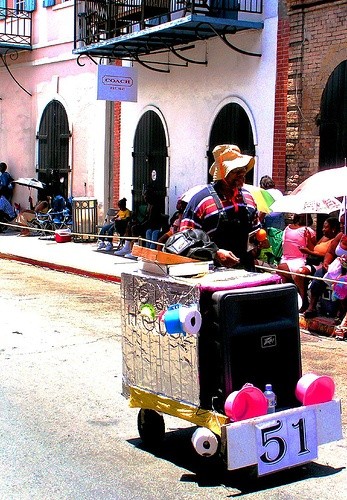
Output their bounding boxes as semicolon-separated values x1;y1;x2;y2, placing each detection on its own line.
0;196;73;239
95;209;123;251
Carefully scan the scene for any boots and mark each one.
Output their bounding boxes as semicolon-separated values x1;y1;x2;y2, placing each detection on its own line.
113;240;139;259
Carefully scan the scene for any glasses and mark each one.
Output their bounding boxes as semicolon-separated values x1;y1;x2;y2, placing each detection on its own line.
231;166;247;173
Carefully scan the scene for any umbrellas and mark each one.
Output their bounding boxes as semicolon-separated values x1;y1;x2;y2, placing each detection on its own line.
178;166;347;235
13;177;44;197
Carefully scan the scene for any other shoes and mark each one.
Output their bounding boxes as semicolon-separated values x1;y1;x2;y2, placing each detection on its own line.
334;325;347;330
304;309;318;317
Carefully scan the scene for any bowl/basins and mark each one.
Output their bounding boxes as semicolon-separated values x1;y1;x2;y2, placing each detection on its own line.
295;374;335;406
225;383;267;421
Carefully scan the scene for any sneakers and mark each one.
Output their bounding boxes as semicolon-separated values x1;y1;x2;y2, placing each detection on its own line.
97;239;113;251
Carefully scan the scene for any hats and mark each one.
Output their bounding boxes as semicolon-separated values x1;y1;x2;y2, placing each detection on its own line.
209;144;255;180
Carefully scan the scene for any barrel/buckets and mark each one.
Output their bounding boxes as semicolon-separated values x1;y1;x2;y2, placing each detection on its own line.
56;229;71;243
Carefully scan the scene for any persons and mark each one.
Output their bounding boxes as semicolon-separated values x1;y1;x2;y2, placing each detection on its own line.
0;162;15;232
97;175;347;329
179;146;270;271
16;192;49;238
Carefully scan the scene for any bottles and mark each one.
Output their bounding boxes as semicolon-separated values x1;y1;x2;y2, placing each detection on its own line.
264;384;277;415
